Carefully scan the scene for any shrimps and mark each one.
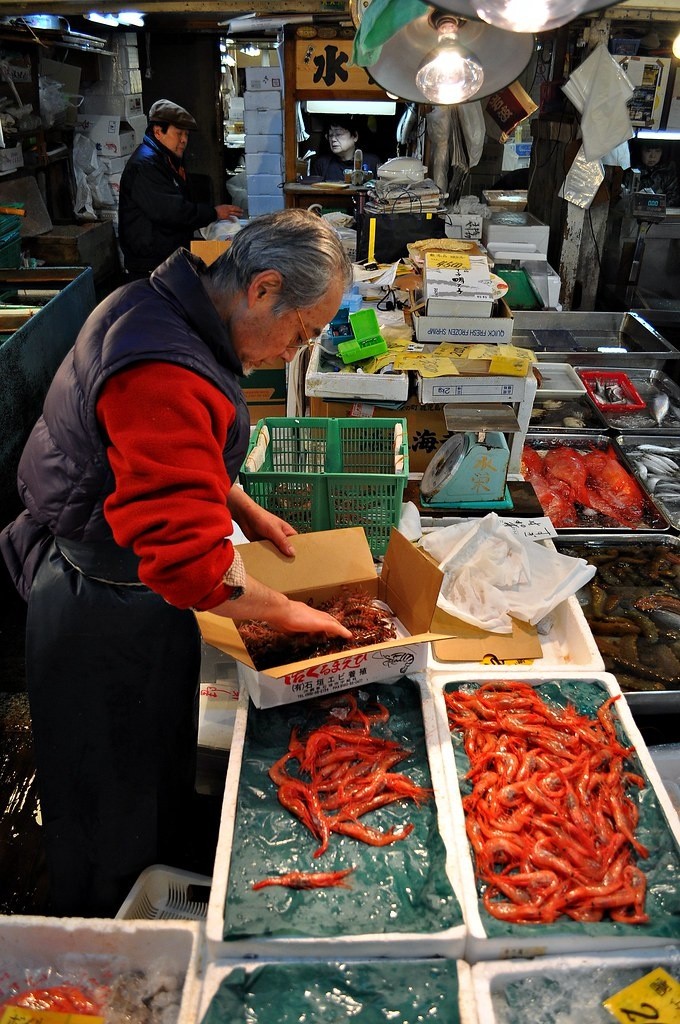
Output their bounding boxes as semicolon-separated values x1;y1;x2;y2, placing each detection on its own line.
252;868;356;892
372;644;420;674
443;681;650;925
267;694;437;858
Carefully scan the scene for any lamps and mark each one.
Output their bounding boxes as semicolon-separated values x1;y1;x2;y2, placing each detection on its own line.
83;12;145;28
418;1;628;33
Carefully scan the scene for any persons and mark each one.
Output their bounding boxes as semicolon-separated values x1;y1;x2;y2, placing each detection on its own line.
115;99;245;276
306;119;383;181
628;141;680;214
2;206;355;913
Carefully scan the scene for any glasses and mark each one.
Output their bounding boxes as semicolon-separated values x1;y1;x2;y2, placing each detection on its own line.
324;130;348;138
286;306;315;350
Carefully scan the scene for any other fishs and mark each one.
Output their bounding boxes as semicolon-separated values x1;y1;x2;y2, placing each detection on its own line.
647;389;670;426
591;374;627;405
521;441;680;530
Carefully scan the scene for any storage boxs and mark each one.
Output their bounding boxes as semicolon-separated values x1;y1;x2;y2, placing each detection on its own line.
1;34;680;1024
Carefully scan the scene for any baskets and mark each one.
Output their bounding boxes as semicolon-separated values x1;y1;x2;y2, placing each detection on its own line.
578;370;646;410
238;417;409;555
112;863;213;927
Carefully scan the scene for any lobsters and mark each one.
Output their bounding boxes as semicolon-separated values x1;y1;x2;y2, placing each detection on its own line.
238;583;397;672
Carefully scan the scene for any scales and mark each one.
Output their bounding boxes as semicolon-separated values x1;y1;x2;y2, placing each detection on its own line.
418;404;522;511
486;241;549;311
619;192;667;313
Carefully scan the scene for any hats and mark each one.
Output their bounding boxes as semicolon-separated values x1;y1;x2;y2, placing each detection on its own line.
149;99;198;127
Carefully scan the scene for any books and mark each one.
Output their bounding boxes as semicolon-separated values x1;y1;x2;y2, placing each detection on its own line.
362;171;453;214
